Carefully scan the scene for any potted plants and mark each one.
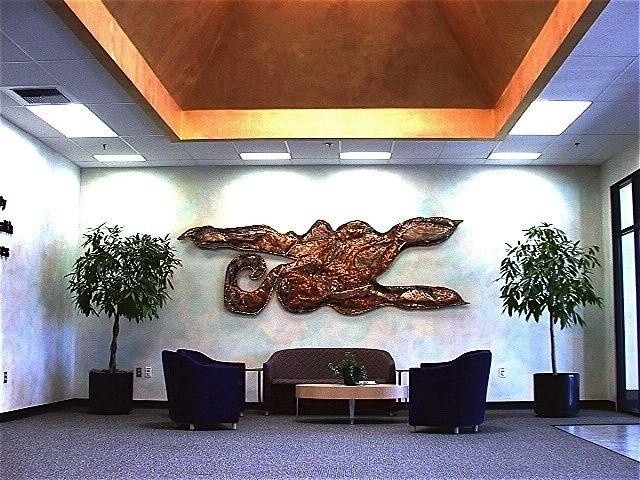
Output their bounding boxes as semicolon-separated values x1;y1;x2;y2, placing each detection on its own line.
327;350;369;386
62;221;183;417
490;221;606;418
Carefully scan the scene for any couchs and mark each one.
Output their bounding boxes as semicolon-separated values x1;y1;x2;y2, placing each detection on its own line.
261;347;398;417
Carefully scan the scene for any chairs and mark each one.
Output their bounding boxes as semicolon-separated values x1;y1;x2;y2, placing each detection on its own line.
408;349;493;435
160;344;248;430
